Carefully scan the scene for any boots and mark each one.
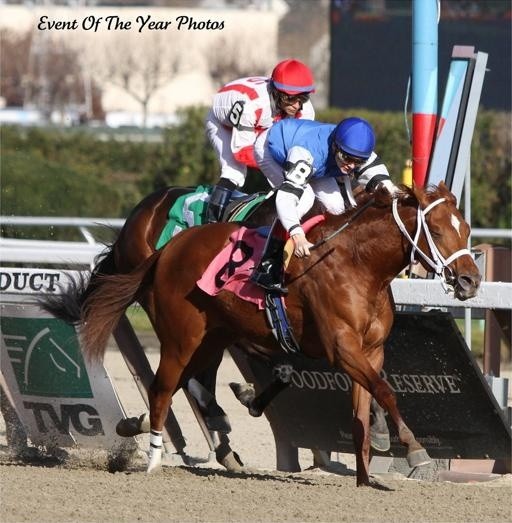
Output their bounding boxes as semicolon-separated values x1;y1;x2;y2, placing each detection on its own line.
251;235;289;295
204;185;232;223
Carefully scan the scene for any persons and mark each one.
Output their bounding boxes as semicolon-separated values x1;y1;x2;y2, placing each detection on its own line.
204;59;316;224
249;116;395;295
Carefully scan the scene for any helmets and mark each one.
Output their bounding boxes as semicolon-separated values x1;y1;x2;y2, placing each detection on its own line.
272;59;316;93
333;118;375;160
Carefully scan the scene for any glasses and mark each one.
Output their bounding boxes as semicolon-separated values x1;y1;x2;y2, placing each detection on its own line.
277;95;310;105
340;150;367;166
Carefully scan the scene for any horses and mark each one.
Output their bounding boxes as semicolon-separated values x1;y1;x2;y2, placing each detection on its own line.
27;178;482;487
